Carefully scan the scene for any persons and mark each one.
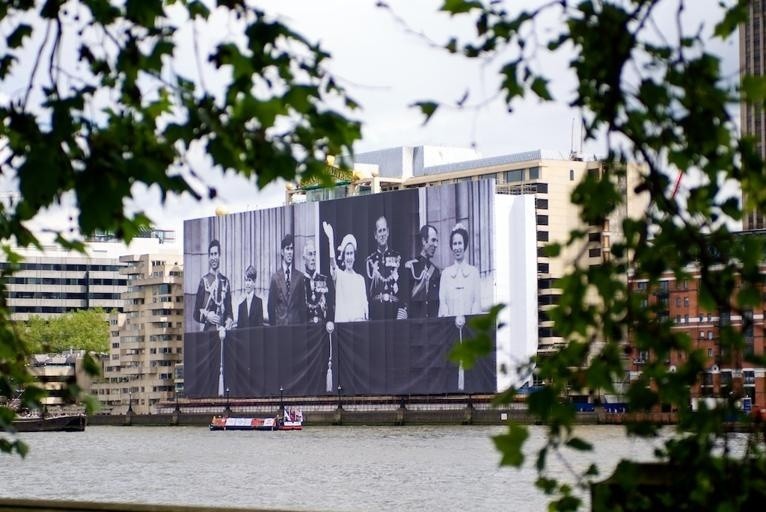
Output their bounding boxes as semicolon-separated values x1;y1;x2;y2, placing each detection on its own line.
438;223;483;318
361;214;404;320
322;220;370;323
236;265;263;329
193;239;233;331
303;240;335;325
397;225;440;320
267;234;308;325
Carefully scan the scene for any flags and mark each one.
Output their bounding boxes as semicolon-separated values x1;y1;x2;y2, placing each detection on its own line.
284;408;303;422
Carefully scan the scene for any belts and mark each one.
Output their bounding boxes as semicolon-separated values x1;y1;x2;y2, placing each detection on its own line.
373;293;399;303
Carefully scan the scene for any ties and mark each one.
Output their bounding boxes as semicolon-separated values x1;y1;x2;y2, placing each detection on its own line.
285;270;291;290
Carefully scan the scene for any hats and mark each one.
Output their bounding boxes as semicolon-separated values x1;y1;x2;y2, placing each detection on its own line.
336;233;358;260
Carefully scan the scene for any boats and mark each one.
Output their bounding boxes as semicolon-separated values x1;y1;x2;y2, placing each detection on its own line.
7;411;87;432
209;409;303;431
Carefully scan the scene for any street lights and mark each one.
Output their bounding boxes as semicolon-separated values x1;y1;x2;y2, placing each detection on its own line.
225;385;230;410
337;385;342;408
278;384;285;409
128;390;132;411
175;388;179;411
